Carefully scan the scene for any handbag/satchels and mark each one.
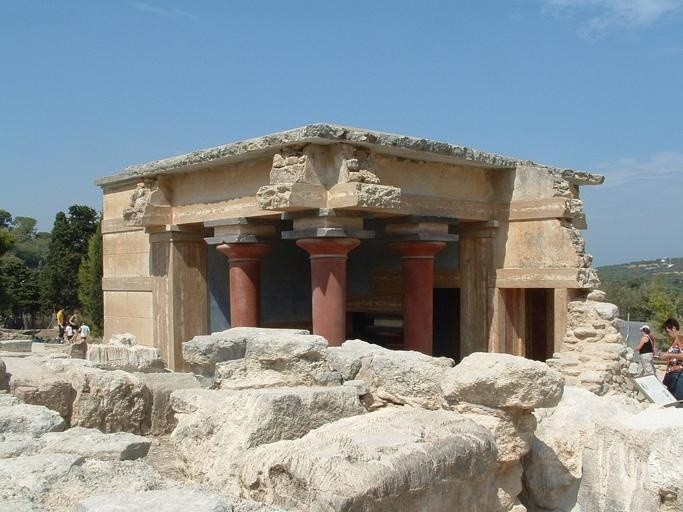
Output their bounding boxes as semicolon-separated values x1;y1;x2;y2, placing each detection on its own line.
663;373;678;391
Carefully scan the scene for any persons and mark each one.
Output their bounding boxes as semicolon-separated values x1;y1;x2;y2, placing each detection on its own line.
633;327;657;378
660;318;682;408
63;324;73;338
54;307;66;342
77;320;90;344
69;312;79;336
